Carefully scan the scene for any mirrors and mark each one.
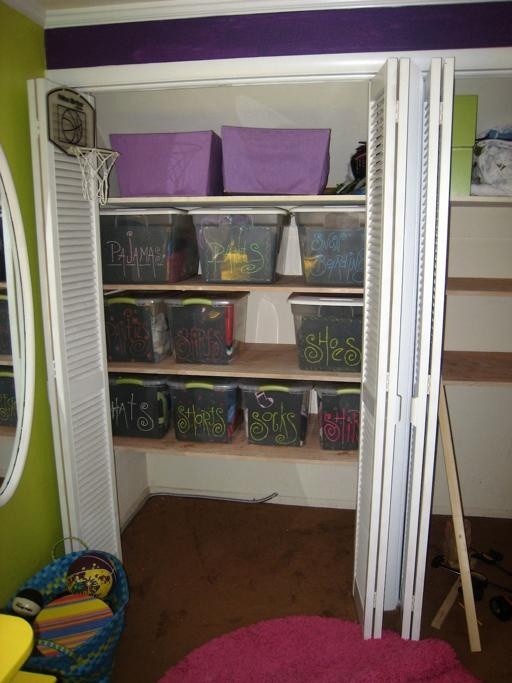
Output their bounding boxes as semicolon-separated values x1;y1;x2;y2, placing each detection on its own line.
0;150;36;506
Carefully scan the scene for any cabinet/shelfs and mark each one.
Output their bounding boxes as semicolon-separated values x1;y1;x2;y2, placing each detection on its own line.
0;280;19;436
96;192;368;464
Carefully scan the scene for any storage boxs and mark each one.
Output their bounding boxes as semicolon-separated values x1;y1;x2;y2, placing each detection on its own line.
104;129;224;195
0;367;17;427
163;288;248;364
165;378;242;444
223;126;332;197
108;372;171;438
188;208;291;283
452;95;478;147
293;207;366;286
442;197;512;383
100;208;200;286
288;291;363;373
313;381;362;449
102;288;173;363
238;379;310;446
450;148;472;197
1;295;14;360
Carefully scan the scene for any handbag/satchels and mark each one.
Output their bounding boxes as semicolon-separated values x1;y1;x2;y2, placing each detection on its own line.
471;138;511;197
3;537;130;682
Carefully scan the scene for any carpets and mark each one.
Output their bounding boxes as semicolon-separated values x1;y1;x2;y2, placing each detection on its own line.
158;614;479;681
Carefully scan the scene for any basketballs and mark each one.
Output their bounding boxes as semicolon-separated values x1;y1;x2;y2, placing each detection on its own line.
13;589;45;619
67;552;114;600
